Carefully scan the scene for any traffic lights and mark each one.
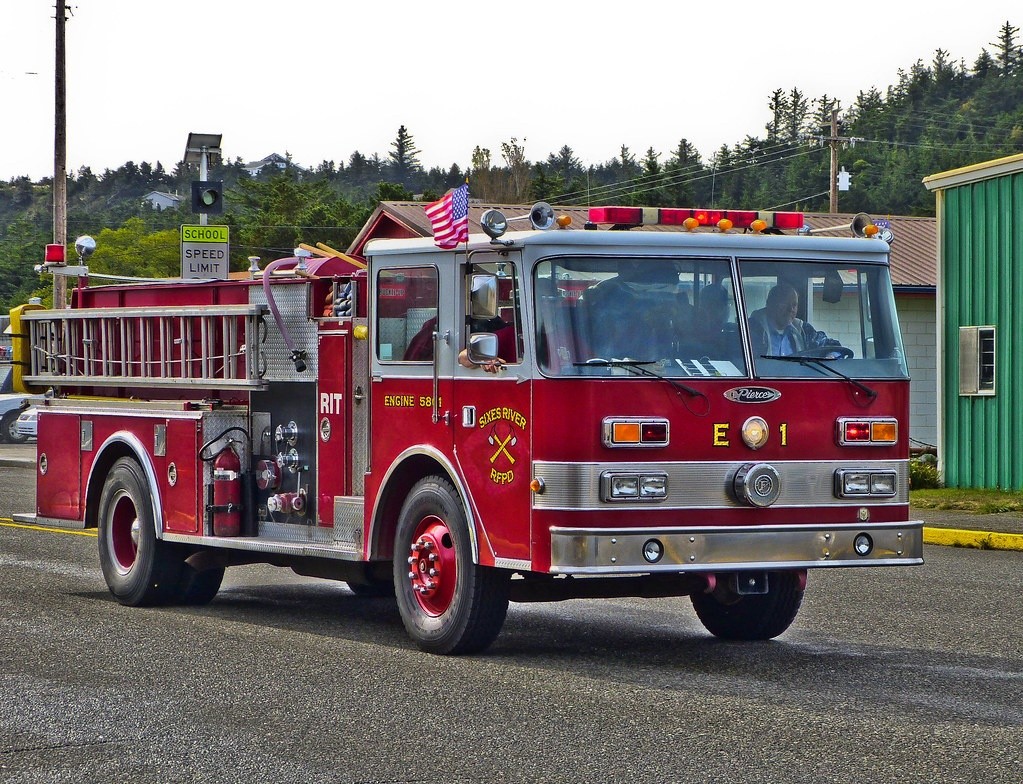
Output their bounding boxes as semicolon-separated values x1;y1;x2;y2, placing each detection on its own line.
190;177;222;213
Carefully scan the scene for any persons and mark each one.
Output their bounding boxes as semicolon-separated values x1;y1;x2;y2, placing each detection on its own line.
578;257;844;362
458;278;592;375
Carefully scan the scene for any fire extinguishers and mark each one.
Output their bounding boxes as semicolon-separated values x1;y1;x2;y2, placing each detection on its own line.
198;427;250;537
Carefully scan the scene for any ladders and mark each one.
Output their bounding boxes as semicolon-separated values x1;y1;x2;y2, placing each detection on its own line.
9;304;270;394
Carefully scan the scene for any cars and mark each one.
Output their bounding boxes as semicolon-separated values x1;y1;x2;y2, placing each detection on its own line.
0;344;13;361
15;407;43;438
0;392;45;443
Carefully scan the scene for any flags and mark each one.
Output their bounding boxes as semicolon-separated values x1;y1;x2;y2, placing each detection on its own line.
425;184;469;249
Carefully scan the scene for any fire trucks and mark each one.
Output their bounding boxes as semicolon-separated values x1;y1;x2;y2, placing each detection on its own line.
2;170;926;657
